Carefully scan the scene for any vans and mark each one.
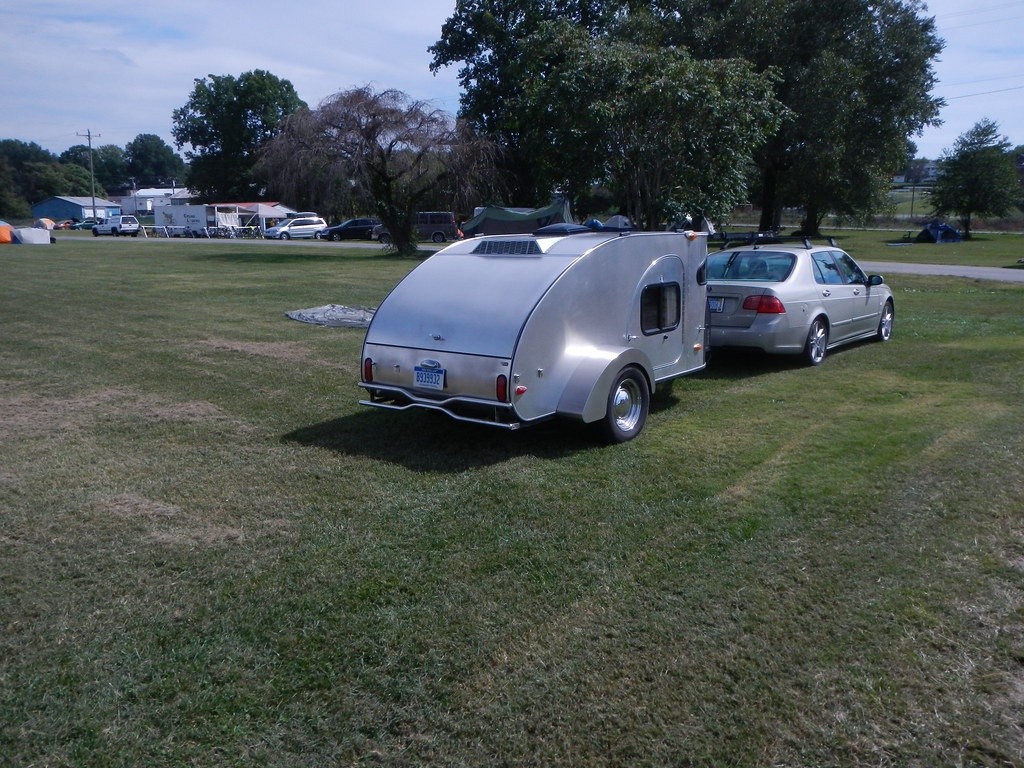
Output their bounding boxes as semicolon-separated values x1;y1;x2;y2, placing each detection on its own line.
371;210;459;244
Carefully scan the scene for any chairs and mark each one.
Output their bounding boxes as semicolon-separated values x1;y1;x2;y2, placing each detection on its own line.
746;258;772;279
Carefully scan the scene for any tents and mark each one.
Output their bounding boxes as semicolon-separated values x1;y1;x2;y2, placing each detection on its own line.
604;215;633;232
458;196;574;236
914;219;961;245
583;219;604;232
666;211;716;236
36;217;56;230
0;220;19;244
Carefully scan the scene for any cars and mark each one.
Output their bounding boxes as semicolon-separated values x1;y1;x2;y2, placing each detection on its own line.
699;241;894;364
53;219;74;231
263;216;330;240
320;217;381;242
70;220;99;230
82;217;104;225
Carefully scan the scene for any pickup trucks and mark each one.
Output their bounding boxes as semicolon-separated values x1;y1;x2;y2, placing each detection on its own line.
92;215;141;238
